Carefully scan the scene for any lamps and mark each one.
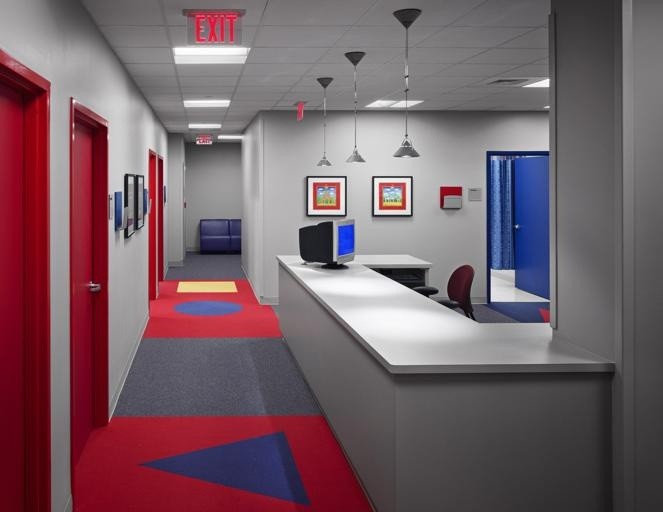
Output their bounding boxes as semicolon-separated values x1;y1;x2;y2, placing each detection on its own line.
344;50;368;166
315;75;334;170
391;10;422;159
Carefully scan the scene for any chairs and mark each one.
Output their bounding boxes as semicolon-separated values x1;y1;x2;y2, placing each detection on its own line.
412;263;477;321
199;218;241;255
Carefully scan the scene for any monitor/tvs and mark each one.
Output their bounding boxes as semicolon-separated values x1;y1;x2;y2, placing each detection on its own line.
298;219;355;269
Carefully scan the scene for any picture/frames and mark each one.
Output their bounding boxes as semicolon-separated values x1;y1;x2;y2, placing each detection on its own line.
124;172;136;238
306;175;348;218
134;175;144;230
371;175;414;218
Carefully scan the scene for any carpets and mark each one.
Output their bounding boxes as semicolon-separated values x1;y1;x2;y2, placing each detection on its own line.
143;278;283;340
481;301;550;322
70;414;375;511
